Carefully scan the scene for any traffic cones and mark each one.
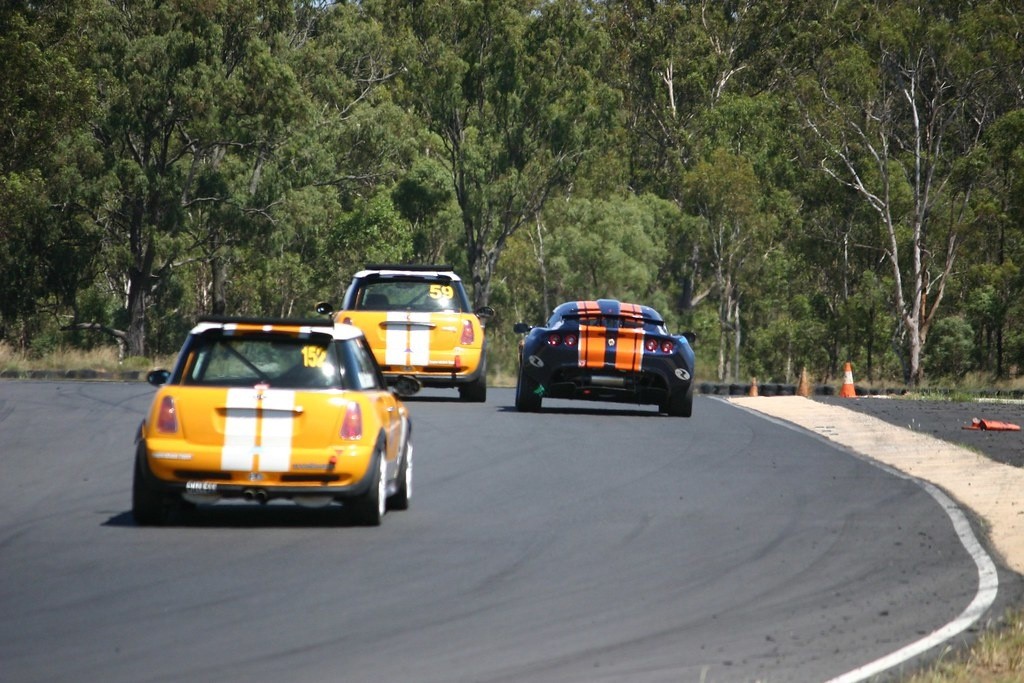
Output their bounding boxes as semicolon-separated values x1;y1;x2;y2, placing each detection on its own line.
748;376;759;396
797;367;813;396
838;361;859;398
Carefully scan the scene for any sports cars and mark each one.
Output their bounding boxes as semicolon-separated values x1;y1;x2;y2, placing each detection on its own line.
513;299;698;417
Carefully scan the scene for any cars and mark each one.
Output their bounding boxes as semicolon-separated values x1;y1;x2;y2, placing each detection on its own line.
314;265;497;401
133;316;422;527
